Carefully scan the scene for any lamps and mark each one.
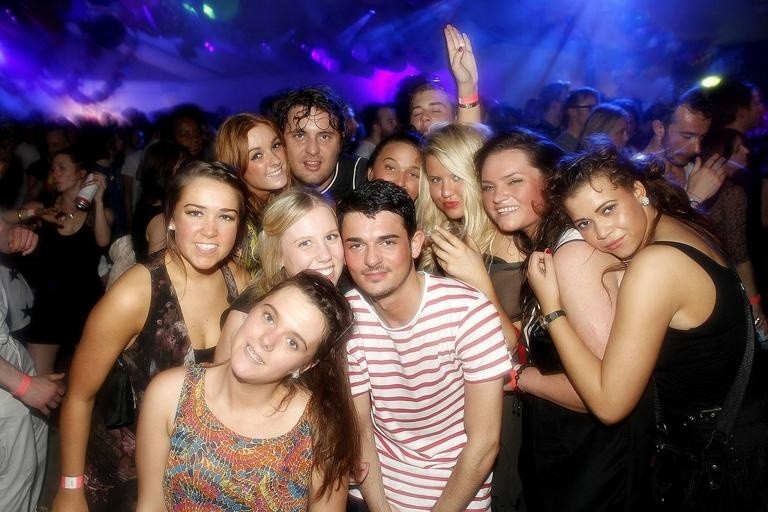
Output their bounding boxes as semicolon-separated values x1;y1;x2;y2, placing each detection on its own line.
181;1;239;22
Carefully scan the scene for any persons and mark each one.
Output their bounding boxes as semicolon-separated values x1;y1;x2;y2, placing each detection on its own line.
0;22;768;512
